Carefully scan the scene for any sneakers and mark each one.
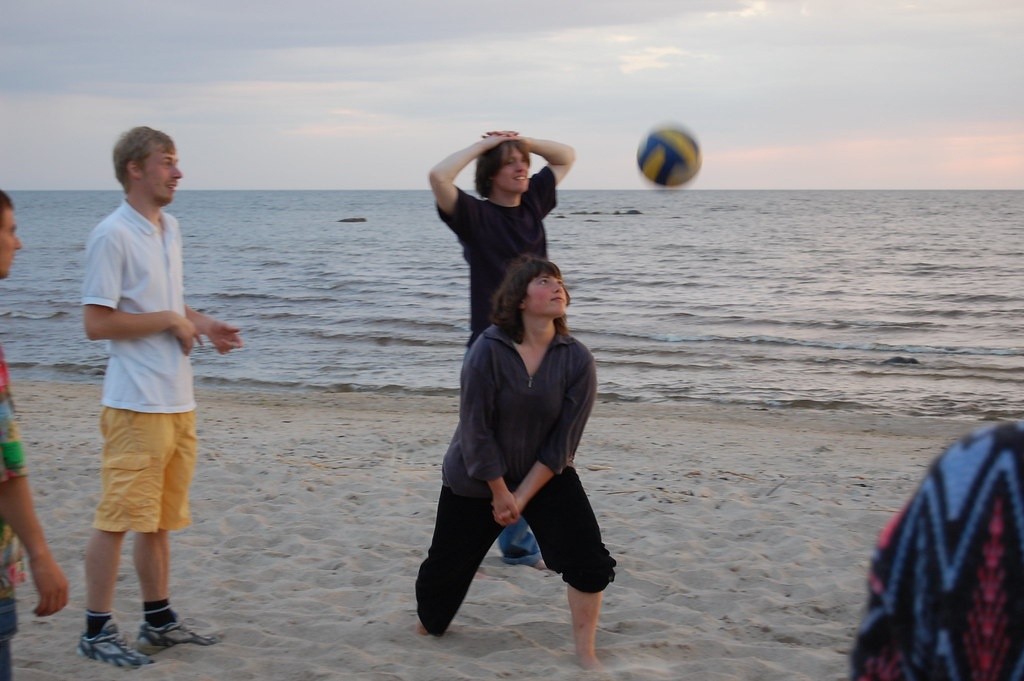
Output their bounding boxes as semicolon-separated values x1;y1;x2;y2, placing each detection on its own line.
137;618;221;655
76;618;155;669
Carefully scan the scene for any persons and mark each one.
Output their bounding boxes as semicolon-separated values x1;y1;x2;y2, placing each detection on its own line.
428;131;575;571
414;256;616;669
0;189;69;681
77;125;243;669
850;420;1023;681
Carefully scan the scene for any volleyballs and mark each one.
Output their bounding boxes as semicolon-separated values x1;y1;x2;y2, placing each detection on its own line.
637;123;704;190
